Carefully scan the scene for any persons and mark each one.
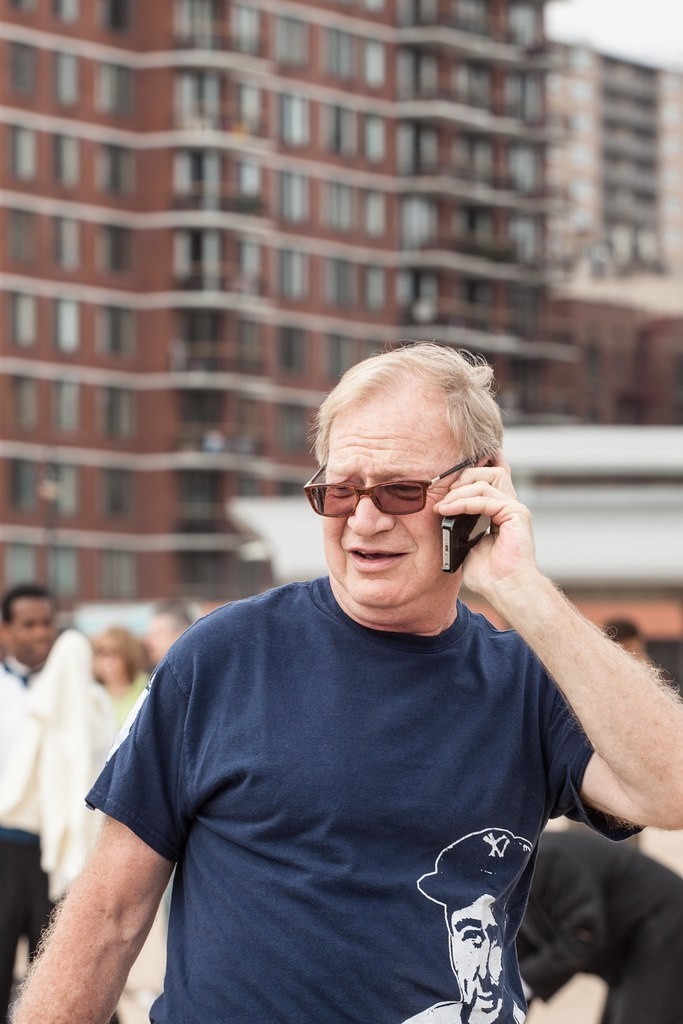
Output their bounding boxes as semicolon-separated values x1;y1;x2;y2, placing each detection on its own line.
8;346;683;1023
1;583;61;1023
516;814;683;1024
603;616;650;666
91;629;151;733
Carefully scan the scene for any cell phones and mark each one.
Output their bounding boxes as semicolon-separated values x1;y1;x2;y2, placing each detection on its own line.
442;508;491;574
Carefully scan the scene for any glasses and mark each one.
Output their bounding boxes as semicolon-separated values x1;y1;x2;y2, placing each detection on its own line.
304;449;488;518
